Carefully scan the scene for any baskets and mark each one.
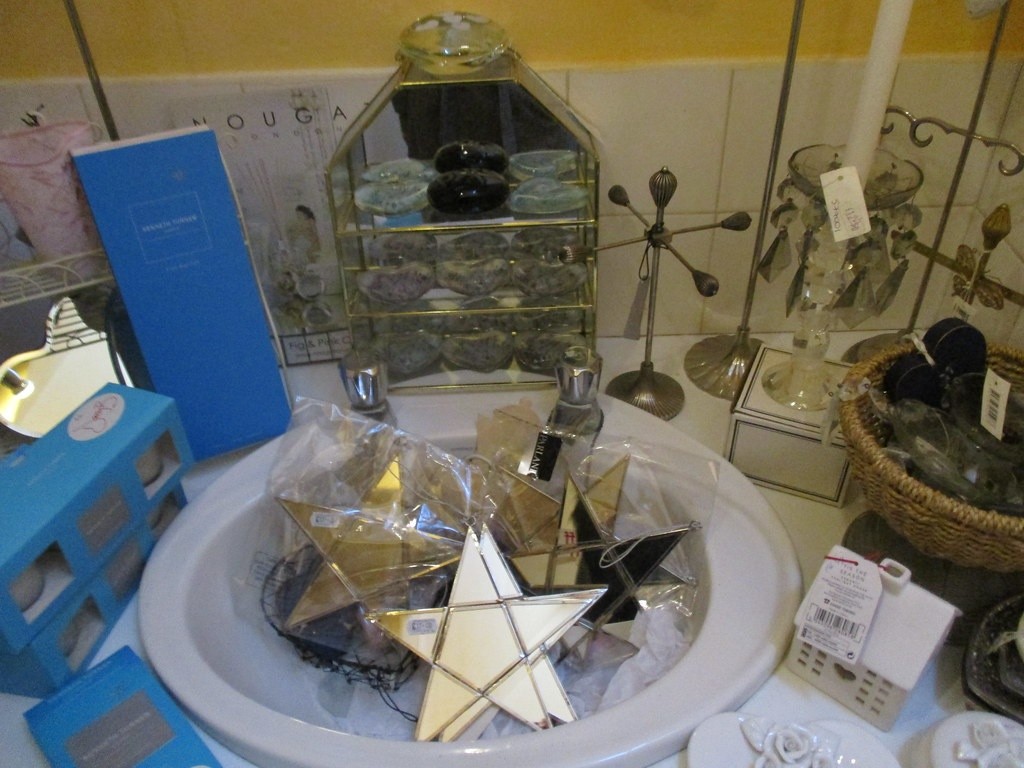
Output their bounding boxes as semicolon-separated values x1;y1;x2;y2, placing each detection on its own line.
838;341;1023;573
